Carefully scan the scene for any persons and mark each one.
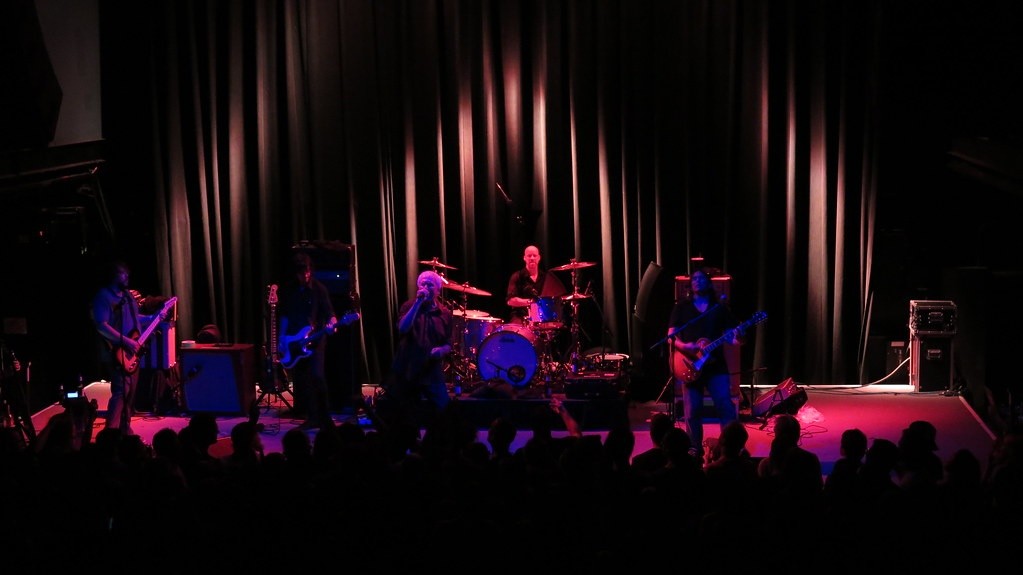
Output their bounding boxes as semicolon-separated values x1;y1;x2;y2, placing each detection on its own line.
92;258;178;434
0;394;1023;575
0;336;29;417
668;270;743;459
278;253;338;427
505;245;566;358
382;271;450;437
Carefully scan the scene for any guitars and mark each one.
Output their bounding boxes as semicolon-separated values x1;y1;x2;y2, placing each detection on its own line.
113;296;177;376
258;284;289;394
278;310;360;370
668;310;769;385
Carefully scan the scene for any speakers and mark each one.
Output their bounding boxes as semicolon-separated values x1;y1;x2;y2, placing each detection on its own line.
303;265;366;413
673;332;742;397
753;377;798;414
182;351;254;415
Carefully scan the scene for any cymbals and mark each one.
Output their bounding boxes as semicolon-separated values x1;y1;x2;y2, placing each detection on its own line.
416;260;458;270
559;294;593;302
440;279;458;288
548;261;597;271
446;284;492;296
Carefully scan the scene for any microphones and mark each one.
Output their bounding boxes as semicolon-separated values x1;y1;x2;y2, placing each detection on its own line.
418;291;425;300
192;364;202;375
585;281;591;295
720;295;728;304
115;297;127;310
514;373;524;381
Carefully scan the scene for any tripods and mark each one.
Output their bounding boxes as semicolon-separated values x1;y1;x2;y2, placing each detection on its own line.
444;292;479;388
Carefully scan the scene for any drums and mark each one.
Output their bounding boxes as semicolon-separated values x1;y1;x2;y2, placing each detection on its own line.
528;296;567;331
593;353;630;394
449;310;489;347
464;317;503;356
477;323;543;387
